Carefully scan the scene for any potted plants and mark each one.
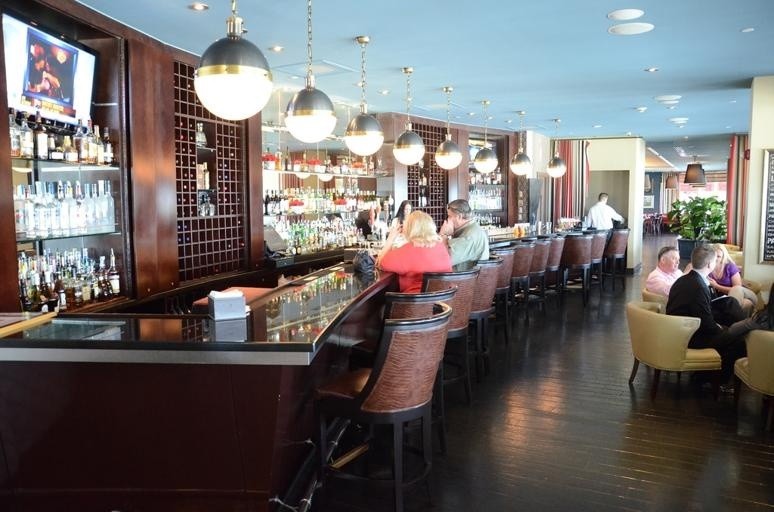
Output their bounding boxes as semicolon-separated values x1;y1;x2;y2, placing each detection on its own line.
668;196;728;259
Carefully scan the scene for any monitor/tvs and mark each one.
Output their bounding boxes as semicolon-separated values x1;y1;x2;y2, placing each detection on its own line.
264;224;289;257
0;8;101;133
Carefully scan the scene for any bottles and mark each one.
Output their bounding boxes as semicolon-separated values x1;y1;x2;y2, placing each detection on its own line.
284;217;382;255
227;245;231;248
239;241;245;246
262;269;354;343
15;178;115;239
196;99;199;104
187;84;191;88
419;158;428;208
559;218;579;230
264;187;381;215
513;224;525;237
545;222;551;230
536;221;541;235
18;247;120;313
264;145;375;177
9;106;114;167
469;167;503;228
215;269;218;273
176;122;241;242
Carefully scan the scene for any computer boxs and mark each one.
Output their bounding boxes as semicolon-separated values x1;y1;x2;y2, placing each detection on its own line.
266;256;296;268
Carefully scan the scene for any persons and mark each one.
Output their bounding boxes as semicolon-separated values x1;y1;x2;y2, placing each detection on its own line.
376;210;453;294
644;242;774;398
438;198;490;272
586;192;626;279
391;200;414;241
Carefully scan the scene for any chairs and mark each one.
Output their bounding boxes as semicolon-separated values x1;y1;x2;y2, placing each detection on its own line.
494;248;515;348
605;228;630;291
644;212;668;236
511;243;536;331
422;266;481;413
565;234;593;308
590;231;608;301
352;276;458;457
546;237;566;313
473;253;504;379
529;239;552;318
625;243;774;433
312;300;456;512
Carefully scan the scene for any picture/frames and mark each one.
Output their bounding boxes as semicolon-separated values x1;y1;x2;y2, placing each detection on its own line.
644;195;654;209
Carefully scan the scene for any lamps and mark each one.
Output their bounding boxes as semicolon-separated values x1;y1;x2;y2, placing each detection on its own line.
342;34;385;156
472;100;499;175
433;86;462;170
666;173;677;189
391;64;427;168
286;0;338;145
684;156;706;188
511;111;530;175
547;118;566;178
191;1;276;125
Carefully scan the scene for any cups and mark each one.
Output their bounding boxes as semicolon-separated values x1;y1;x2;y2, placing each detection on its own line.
481;225;512;234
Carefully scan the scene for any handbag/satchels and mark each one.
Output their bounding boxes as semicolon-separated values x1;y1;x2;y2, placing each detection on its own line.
353;251;375;273
709;285;739;308
729;308;770;336
